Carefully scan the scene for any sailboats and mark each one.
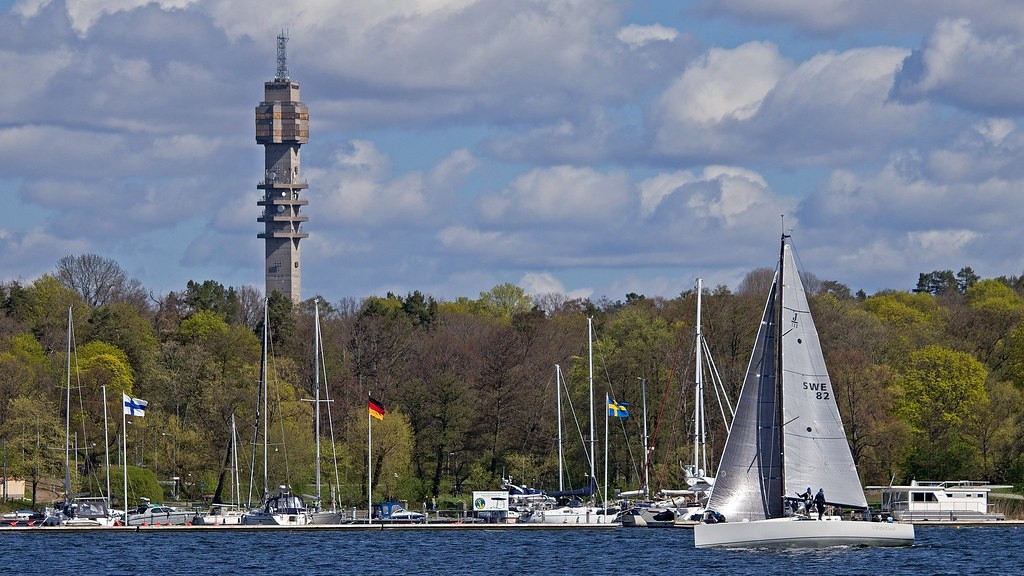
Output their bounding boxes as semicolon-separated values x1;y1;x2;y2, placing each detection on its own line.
691;213;917;552
1;276;837;530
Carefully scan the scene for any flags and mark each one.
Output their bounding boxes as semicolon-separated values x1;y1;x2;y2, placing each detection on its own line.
608;397;630;418
368;396;386;421
124;393;149;418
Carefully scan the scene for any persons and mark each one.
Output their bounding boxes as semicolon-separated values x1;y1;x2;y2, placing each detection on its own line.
813;488;827;520
795;487;814;518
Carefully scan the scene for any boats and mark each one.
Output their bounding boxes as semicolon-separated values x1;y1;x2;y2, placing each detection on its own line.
850;480;1007;525
